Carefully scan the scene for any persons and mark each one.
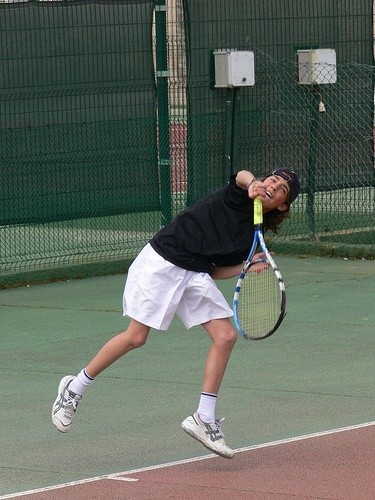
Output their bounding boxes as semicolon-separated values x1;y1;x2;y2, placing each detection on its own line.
50;167;304;460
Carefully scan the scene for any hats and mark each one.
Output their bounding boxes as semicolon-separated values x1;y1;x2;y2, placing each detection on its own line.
271;165;300;204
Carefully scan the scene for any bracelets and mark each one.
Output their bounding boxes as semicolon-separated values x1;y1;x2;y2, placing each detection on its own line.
247;177;258;191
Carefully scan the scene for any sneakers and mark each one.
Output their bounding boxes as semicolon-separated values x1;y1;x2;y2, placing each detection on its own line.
181;412;236;458
51;375;82;431
